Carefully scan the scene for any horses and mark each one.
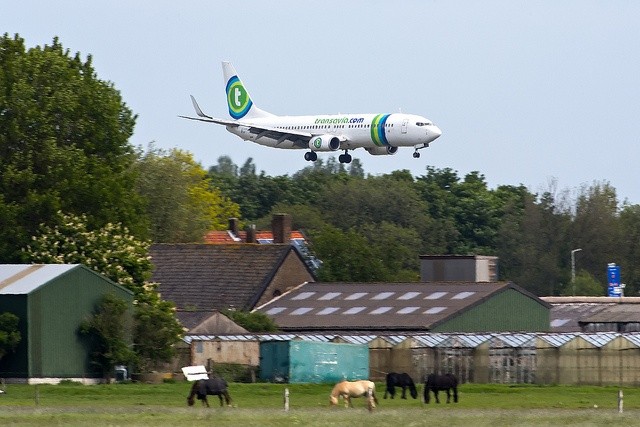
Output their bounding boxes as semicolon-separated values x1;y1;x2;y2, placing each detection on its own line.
330;380;378;412
384;373;417;400
423;374;460;405
187;378;232;410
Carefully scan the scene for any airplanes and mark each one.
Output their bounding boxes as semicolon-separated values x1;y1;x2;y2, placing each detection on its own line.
177;61;441;164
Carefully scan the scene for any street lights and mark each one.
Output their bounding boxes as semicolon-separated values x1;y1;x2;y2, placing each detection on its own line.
570;248;582;287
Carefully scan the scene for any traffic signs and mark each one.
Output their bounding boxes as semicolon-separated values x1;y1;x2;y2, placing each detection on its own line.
607;267;621;295
